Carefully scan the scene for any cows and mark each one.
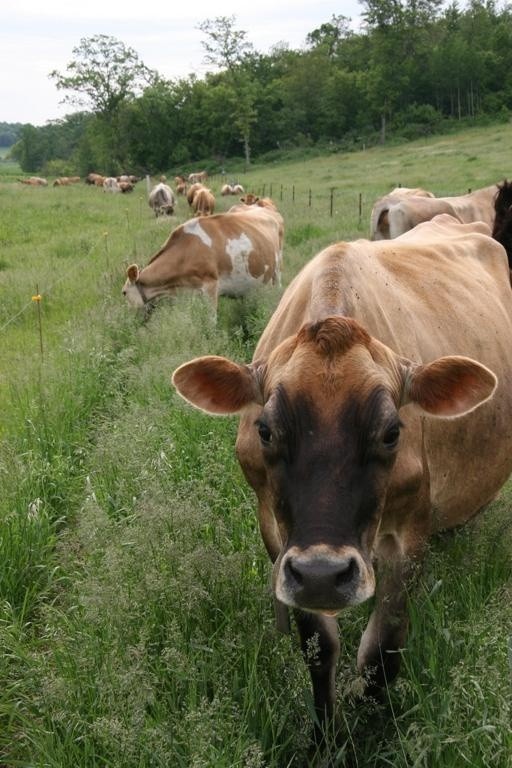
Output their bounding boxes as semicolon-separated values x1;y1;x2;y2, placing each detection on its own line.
148;171;216;217
222;184;243;196
370;181;505;241
239;193;278;211
121;204;284;341
16;173;138;195
172;212;512;768
